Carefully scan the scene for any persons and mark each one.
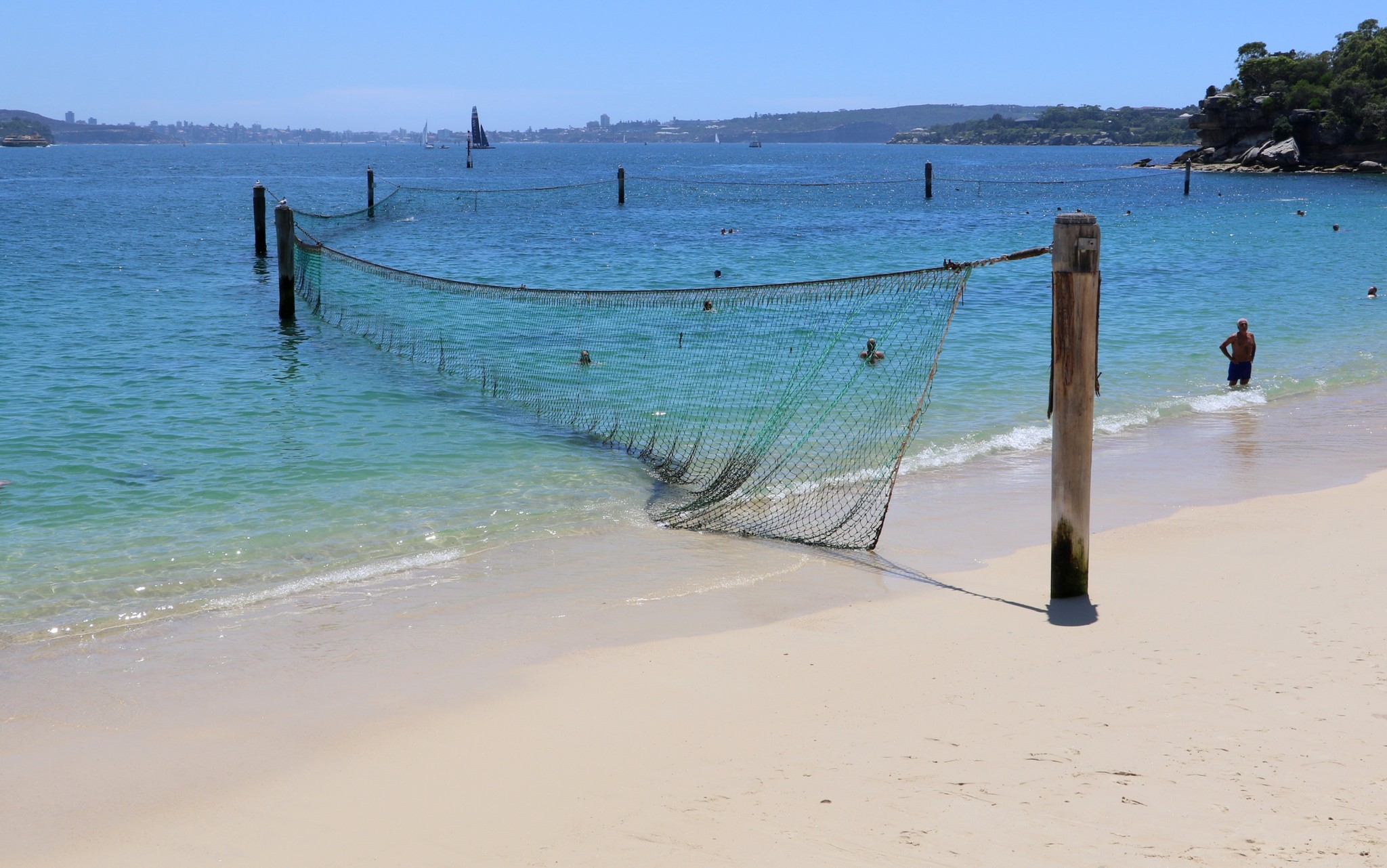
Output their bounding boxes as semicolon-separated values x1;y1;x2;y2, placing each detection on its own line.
719;228;727;234
1333;224;1350;232
714;269;724;278
1299;211;1304;217
1219;318;1256;387
1289;209;1301;215
1077;208;1081;213
860;338;885;359
1367;286;1380;299
702;300;714;312
727;228;735;234
1057;207;1061;211
576;350;592;365
1123;210;1131;216
1026;211;1030;214
1219;193;1222;196
